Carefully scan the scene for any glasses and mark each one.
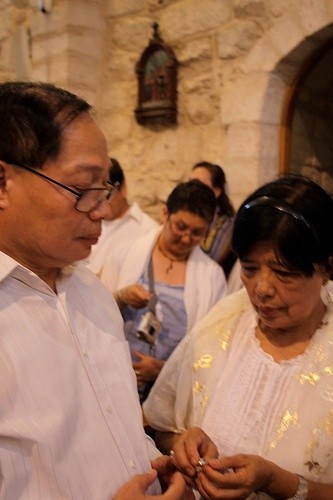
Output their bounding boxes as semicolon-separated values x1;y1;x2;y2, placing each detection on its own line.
18;163;115;211
168;217;208;242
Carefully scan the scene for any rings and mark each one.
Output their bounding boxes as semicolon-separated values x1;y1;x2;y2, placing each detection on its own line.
197;455;208;470
169;449;179;462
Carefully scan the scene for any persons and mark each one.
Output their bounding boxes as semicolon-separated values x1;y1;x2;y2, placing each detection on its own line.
142;177;333;500
189;160;238;283
75;158;163;296
0;79;198;500
113;178;228;403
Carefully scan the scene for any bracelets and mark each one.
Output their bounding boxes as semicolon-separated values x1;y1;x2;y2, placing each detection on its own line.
292;474;308;500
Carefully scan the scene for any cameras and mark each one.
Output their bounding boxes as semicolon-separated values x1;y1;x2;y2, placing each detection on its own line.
135;311;160;344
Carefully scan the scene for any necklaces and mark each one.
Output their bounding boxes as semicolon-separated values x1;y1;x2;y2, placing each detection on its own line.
156;243;190;273
